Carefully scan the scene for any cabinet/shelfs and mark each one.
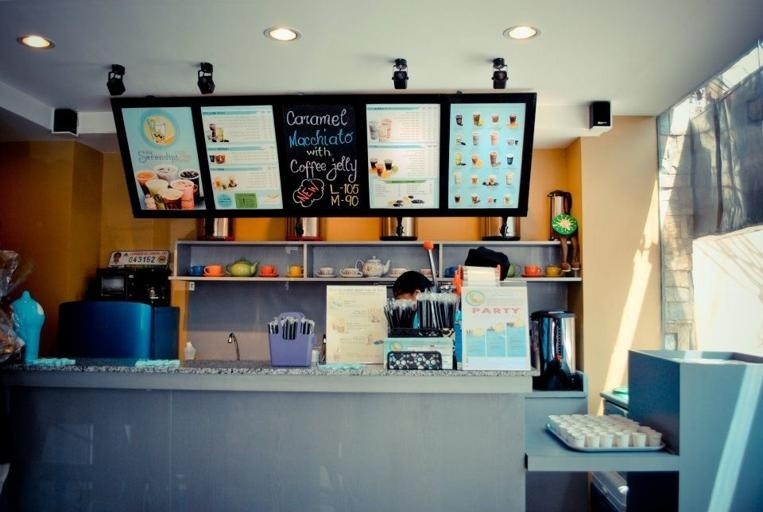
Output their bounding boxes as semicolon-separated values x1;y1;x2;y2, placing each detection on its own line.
167;240;582;360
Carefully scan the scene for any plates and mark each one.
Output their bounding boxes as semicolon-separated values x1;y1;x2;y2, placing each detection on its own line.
258;273;305;278
186;272;224;277
317;272;363;278
521;272;564;278
389;275;434;280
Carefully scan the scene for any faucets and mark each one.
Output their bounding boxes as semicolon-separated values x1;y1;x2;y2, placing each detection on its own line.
227;332;241;361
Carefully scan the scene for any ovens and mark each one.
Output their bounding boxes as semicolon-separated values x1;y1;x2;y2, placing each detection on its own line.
83;264;173;308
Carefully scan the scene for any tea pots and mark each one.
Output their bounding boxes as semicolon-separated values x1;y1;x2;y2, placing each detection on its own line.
225;257;258;277
507;263;520;278
355;255;391;278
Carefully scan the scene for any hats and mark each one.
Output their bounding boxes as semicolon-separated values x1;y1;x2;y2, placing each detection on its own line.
392;269;436;298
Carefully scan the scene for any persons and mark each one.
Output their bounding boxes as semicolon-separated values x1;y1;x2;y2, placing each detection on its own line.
110;251;122;266
393;269;461;366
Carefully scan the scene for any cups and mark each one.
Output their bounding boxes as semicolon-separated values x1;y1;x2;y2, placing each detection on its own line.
319;266;363;274
390;267;432;278
523;264;562;275
261;263;304;274
138;164;201;210
445;267;457;278
188;264;226;274
542;412;665;451
